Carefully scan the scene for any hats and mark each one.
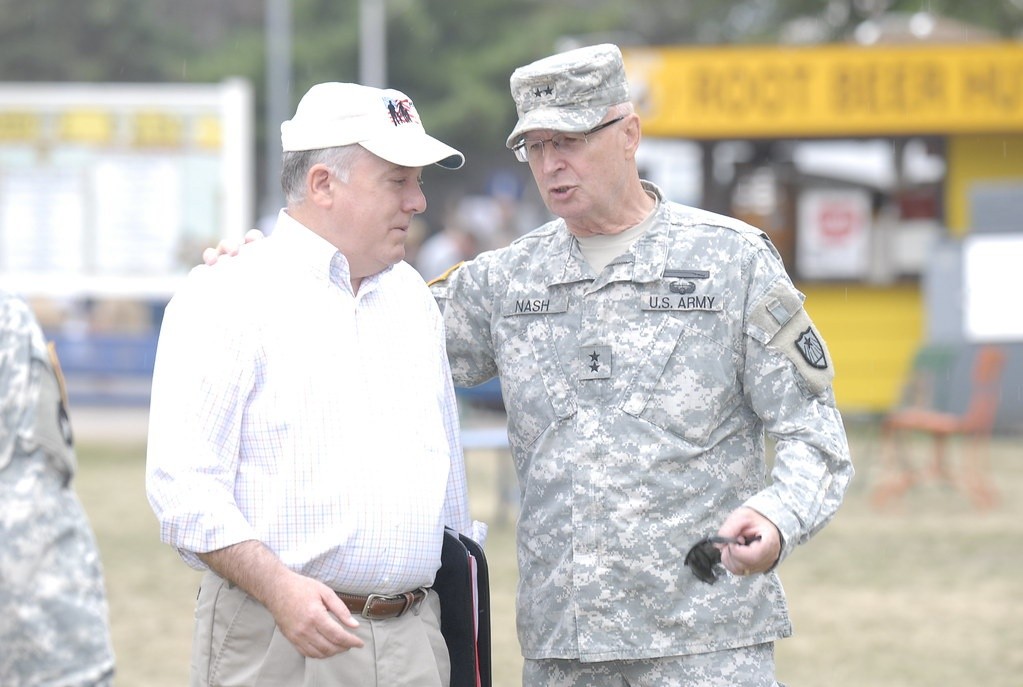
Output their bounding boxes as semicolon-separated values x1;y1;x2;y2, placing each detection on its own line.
280;81;467;169
506;42;629;149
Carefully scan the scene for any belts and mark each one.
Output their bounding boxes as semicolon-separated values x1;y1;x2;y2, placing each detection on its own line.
336;589;430;620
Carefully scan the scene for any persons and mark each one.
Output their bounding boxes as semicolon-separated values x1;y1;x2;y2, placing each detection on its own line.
1;296;117;687
146;80;486;687
203;43;855;687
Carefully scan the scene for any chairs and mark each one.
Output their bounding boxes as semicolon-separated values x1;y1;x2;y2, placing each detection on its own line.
880;346;1007;507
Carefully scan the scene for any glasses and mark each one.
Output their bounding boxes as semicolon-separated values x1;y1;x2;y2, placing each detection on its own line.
512;116;624;163
685;538;741;587
